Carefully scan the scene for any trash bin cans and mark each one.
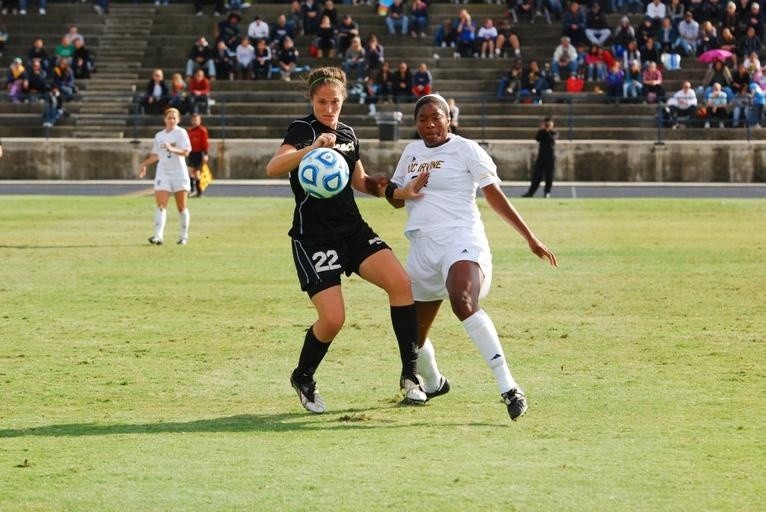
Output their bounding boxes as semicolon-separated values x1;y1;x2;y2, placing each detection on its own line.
375;111;403;140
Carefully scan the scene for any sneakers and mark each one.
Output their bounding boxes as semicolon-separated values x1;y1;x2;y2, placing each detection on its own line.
502;387;529;422
402;375;451;404
176;239;187;245
187;191;205;198
400;376;427;402
521;191;552;198
148;236;164;245
289;368;326;415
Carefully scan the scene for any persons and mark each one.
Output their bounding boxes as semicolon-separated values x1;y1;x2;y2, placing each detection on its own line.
186;112;209;197
138;107;193;244
266;67;430;414
364;94;558;421
522;117;559;198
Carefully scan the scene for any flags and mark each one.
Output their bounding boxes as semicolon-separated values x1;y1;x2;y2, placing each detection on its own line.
198;153;212;192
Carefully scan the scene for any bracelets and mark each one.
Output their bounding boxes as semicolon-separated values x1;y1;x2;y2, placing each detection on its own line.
385;183;397;199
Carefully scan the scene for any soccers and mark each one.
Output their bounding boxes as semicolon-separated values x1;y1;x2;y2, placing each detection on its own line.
298;148;350;199
